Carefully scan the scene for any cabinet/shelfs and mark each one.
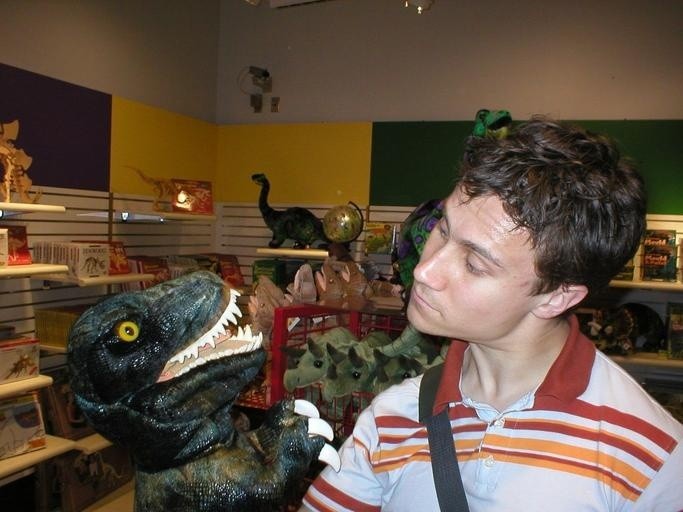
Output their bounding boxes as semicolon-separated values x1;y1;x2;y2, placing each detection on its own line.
0;201;75;478
589;280;683;368
31;271;153;511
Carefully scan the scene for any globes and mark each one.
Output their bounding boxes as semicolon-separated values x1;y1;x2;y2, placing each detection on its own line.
322;201;364;252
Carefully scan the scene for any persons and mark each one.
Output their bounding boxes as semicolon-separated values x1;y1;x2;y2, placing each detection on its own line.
294;109;683;512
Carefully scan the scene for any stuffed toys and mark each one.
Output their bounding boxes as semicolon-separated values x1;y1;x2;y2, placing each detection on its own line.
282;325;448;421
245;256;401;349
